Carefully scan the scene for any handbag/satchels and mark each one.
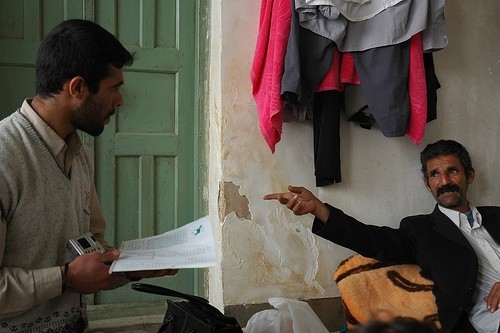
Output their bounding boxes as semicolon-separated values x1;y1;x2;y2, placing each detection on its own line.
130;282;245;333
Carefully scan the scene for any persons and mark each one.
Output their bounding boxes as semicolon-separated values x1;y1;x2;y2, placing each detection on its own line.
263;139;500;333
356;310;439;333
0;19;180;333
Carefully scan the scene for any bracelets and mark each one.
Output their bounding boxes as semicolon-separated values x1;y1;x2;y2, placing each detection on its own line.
62;263;69;293
